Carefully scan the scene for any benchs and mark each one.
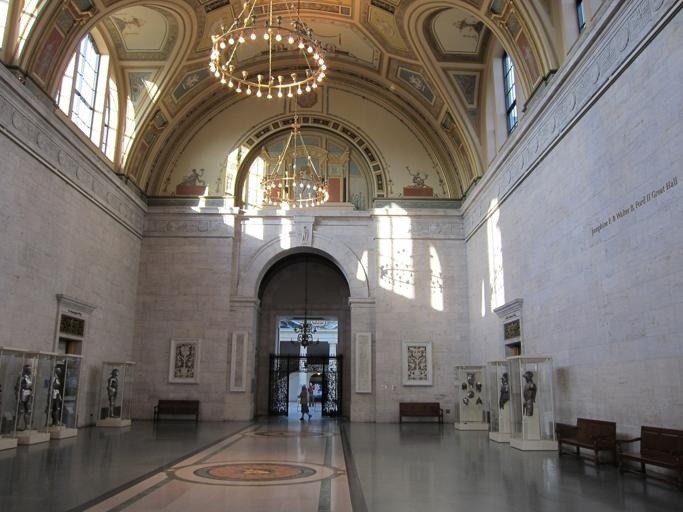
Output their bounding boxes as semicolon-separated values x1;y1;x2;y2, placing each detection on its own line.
400;402;443;424
154;400;200;424
615;425;682;486
555;418;617;468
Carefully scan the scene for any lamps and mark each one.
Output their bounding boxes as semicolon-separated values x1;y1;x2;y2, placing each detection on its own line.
259;106;327;210
290;262;320;347
206;0;327;102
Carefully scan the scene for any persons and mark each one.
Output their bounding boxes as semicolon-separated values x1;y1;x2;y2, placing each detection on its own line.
44;366;62;426
307;382;315;407
520;369;537;416
406;167;424;187
13;364;32;431
296;383;313;421
105;368;119;417
182;168;206;186
496;371;511;408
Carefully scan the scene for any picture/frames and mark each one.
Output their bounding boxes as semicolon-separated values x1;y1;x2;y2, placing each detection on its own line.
168;338;199;384
401;342;433;387
